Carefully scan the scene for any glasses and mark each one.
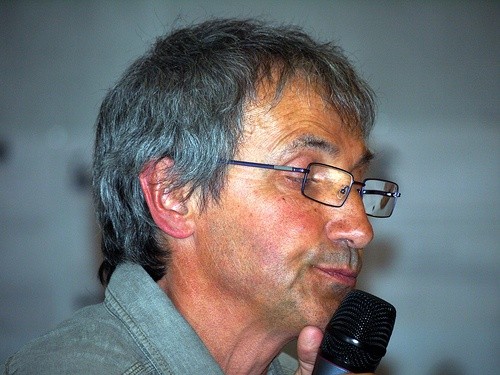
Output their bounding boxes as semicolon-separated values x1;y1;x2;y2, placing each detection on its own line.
220;142;401;218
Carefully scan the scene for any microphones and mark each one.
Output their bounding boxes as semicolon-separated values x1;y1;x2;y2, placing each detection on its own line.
312;290;397;375
